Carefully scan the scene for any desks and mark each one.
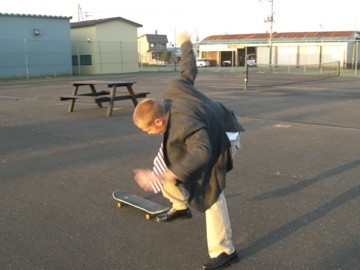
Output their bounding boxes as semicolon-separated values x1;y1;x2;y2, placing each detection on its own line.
68;80;137;117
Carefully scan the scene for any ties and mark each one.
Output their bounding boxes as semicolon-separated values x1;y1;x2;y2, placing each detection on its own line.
148;143;165;194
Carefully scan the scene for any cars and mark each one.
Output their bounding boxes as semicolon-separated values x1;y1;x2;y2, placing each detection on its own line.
196;58;210;67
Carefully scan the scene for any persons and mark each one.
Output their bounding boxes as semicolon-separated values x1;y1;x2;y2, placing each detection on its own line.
132;31;245;270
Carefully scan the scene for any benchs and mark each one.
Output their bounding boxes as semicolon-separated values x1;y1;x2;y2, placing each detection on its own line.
60;91;150;102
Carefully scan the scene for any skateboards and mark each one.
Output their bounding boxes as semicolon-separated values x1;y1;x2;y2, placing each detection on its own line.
112;190;170;219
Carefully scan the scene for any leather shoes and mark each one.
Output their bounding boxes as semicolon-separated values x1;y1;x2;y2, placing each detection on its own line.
154;208;192;224
201;251;239;269
